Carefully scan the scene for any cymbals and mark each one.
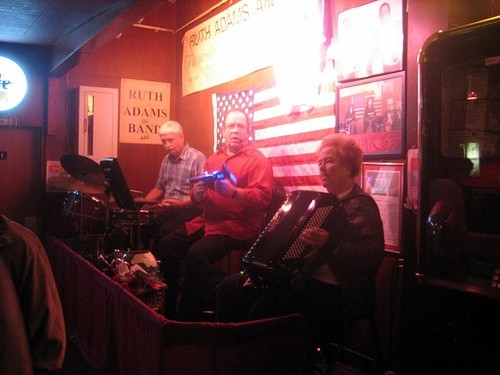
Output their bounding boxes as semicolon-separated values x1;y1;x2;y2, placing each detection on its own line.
111;196;160;204
60;154;111;189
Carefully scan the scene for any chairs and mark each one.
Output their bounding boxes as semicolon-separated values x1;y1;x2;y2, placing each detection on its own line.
219;181;288;274
308;248;383;375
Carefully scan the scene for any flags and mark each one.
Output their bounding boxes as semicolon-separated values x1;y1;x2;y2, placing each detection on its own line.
352;95;382;120
212;68;336;198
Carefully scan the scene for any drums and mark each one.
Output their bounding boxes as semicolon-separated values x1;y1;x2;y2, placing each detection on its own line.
111;207;155;227
61;190;107;219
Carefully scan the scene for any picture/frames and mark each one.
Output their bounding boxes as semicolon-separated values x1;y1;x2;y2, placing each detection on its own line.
359;160;406;254
336;0;406;161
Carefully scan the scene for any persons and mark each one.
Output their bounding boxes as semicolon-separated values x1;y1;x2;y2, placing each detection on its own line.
344;104;357;134
0;215;67;370
376;3;401;72
217;133;384;338
133;122;209;250
152;110;274;319
380;103;398;131
363;96;377;133
